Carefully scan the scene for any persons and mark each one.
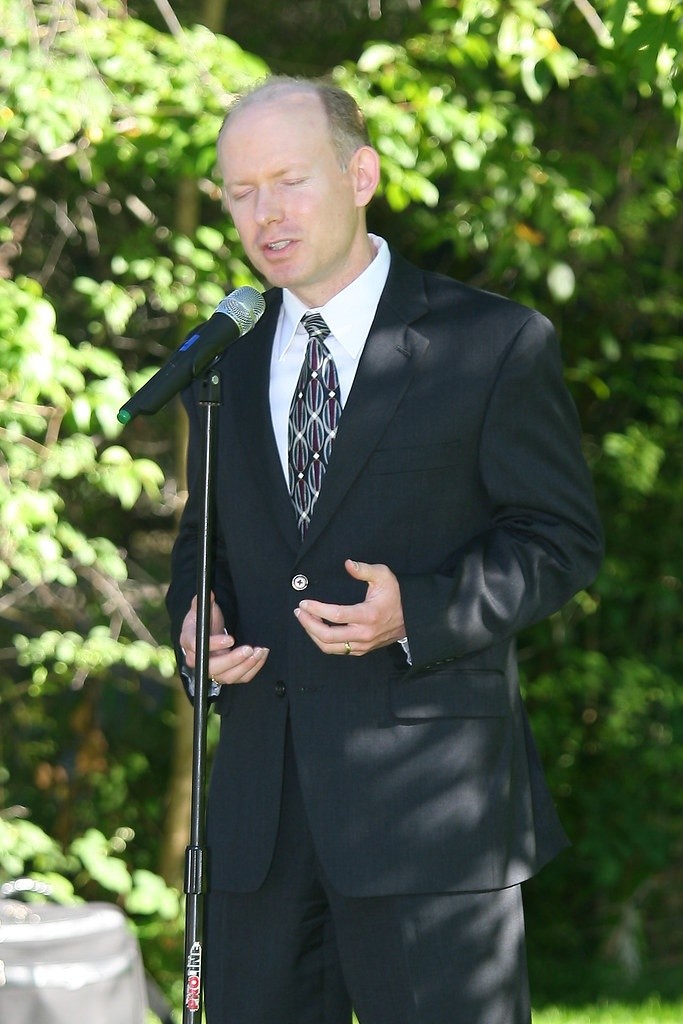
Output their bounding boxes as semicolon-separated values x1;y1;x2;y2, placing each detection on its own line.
165;74;604;1024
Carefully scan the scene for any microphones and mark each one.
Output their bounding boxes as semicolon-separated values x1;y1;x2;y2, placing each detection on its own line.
117;285;266;425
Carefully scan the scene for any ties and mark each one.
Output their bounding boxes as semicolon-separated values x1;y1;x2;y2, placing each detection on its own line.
288;314;343;540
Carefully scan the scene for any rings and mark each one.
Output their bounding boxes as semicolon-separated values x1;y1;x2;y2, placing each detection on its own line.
211;675;222;686
344;642;352;657
